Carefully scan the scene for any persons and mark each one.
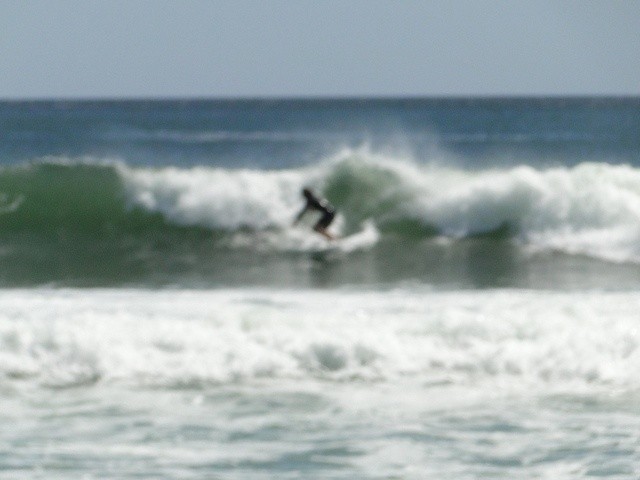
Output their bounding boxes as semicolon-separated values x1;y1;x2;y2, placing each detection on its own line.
292;186;340;241
